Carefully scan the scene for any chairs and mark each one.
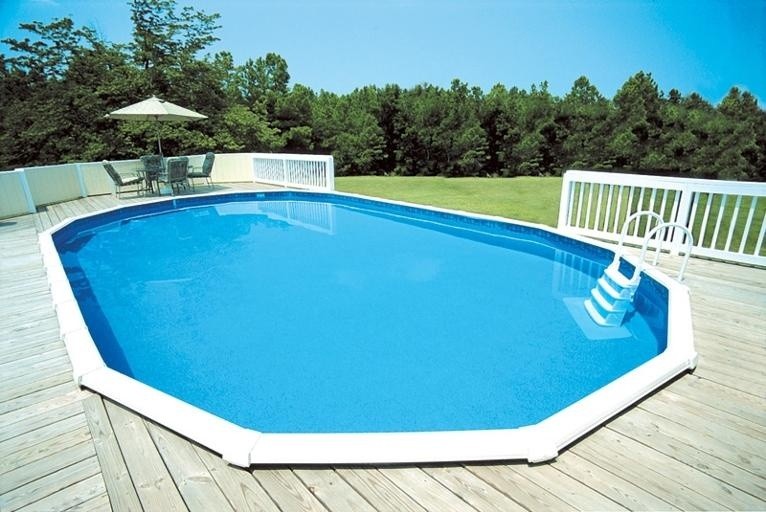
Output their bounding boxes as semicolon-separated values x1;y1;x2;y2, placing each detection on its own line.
102;152;216;197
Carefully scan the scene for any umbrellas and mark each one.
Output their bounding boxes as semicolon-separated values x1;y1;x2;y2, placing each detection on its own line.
106;95;208;155
